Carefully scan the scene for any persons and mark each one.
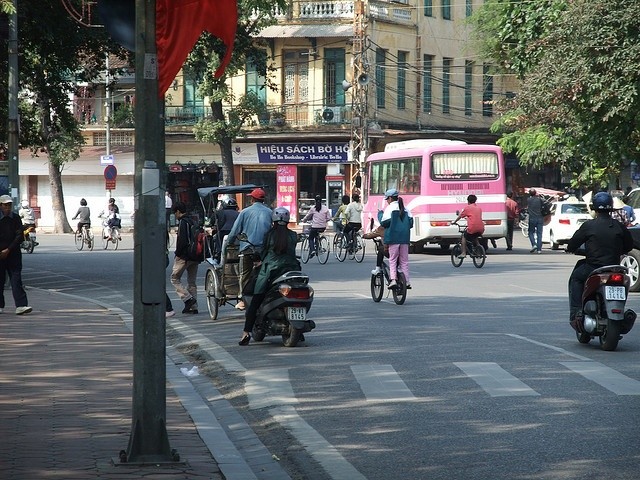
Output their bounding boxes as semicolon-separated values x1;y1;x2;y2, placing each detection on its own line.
73;199;91;249
211;199;238;253
451;195;485;258
165;229;175;318
332;195;350;236
524;190;549;254
344;194;363;249
0;195;33;315
104;207;122;239
621;195;636;222
225;187;273;309
238;207;301;345
19;200;36;241
506;191;524;250
611;197;629;226
300;195;330;259
99;198;122;240
170;203;199;313
567;191;633;329
378;189;414;289
362;225;389;276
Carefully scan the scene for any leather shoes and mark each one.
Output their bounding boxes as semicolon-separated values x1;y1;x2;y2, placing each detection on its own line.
507;248;512;250
508;245;512;250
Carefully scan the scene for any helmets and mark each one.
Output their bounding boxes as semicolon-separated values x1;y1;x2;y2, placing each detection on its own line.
21;201;29;209
272;208;290;225
593;192;613;209
228;198;237;206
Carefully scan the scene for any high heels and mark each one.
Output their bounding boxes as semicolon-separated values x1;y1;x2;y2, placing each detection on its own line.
239;334;251;345
299;334;305;340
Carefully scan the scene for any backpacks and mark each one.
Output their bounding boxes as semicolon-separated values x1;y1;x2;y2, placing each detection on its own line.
178;216;213;263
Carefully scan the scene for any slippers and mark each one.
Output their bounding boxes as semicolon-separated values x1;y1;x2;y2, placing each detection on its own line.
235;302;245;310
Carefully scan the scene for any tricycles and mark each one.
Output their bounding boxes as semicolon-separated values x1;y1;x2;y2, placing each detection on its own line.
203;224;242;320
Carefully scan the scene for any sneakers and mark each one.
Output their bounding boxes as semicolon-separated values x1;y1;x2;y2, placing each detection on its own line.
166;310;176;318
406;283;409;286
189;310;198;314
387;282;397;288
538;251;542;254
0;308;4;313
16;306;32;315
530;245;538;252
182;297;197;312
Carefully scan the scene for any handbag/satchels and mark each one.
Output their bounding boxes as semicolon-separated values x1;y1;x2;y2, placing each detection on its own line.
540;198;548;215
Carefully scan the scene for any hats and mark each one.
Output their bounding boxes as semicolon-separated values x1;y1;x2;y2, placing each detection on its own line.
0;195;13;204
246;188;266;200
384;189;398;200
612;197;625;209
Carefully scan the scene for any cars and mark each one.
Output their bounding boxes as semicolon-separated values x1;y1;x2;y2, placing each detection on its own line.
535;201;594;250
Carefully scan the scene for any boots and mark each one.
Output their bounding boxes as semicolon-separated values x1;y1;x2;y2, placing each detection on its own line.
371;266;381;275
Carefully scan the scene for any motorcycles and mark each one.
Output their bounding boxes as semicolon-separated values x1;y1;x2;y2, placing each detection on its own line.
565;243;637;352
235;232;316;347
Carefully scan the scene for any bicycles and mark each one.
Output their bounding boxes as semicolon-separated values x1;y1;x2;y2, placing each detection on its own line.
300;218;330;265
361;234;412;305
72;217;94;251
335;217;366;263
450;222;488;269
98;216;118;252
328;218;356;260
20;223;40;254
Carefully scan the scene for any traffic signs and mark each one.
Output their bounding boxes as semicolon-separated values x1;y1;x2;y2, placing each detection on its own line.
104;165;117;190
